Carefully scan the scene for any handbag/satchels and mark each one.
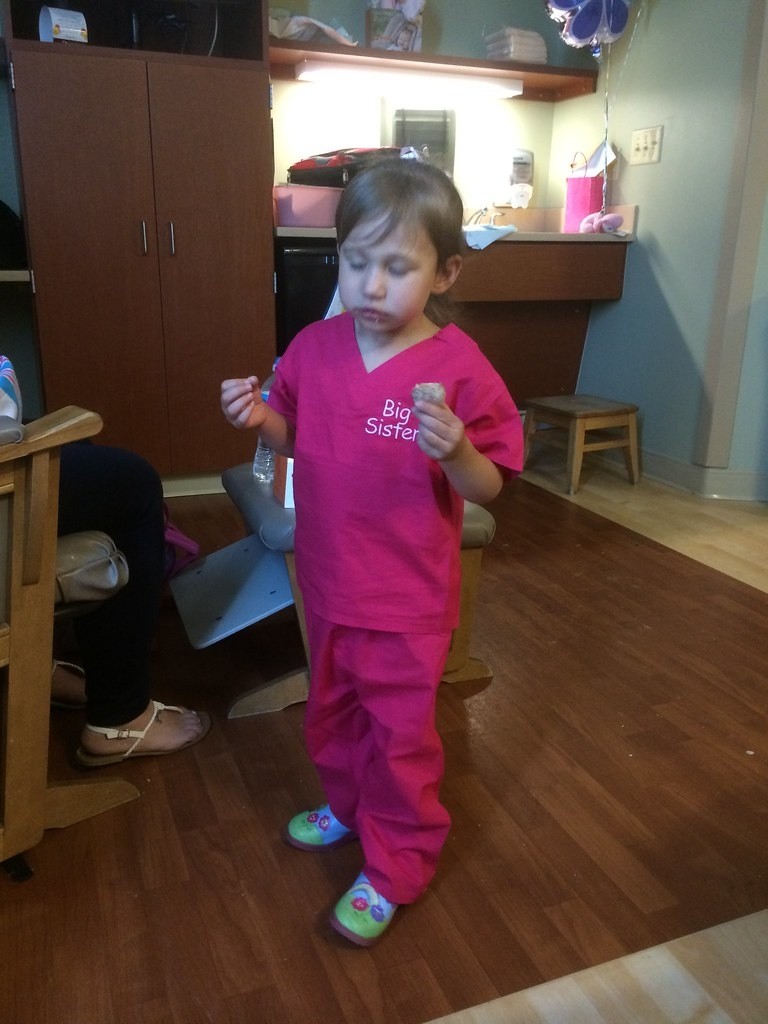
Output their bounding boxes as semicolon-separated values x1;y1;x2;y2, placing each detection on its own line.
564;152;604;234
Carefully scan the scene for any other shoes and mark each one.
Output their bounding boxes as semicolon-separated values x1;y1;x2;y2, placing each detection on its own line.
329;873;400;945
287;802;356;851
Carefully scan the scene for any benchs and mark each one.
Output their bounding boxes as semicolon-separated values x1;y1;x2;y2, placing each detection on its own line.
221;460;497;720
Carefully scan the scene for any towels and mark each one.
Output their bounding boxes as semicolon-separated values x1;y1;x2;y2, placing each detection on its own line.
462;224;517;250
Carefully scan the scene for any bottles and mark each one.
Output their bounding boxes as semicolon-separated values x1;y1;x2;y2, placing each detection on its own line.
253;357;282;483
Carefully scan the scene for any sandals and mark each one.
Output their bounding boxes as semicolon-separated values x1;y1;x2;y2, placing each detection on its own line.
51;659;87;708
77;701;210;765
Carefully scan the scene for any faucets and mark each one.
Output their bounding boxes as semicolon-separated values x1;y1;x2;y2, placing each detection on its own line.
466;207;488;224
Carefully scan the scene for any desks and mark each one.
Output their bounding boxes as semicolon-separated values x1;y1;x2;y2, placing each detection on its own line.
424;231;631;434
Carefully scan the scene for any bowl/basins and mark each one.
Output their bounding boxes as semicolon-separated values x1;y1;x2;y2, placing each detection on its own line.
273;185;343;228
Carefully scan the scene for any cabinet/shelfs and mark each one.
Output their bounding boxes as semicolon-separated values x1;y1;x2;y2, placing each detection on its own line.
272;184;345;228
5;37;279;480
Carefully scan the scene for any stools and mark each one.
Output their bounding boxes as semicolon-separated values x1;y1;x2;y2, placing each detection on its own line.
521;395;641;495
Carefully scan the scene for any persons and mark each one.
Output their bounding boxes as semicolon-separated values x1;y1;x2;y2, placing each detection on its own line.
220;160;526;949
388;28;413;52
1;353;214;767
